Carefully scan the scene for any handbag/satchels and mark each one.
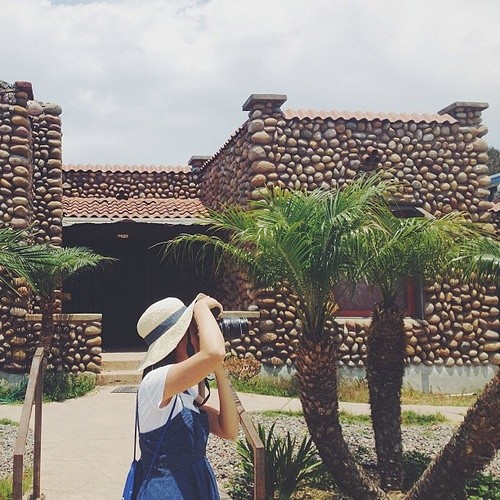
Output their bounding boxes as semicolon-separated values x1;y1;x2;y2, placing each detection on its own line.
121;459;143;500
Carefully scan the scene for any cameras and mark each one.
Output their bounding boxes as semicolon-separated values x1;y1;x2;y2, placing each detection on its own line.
210;305;249;342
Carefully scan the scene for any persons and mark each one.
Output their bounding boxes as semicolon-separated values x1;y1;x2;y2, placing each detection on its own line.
123;293;239;499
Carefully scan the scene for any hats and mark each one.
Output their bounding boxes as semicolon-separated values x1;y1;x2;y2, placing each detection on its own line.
136;293;207;374
